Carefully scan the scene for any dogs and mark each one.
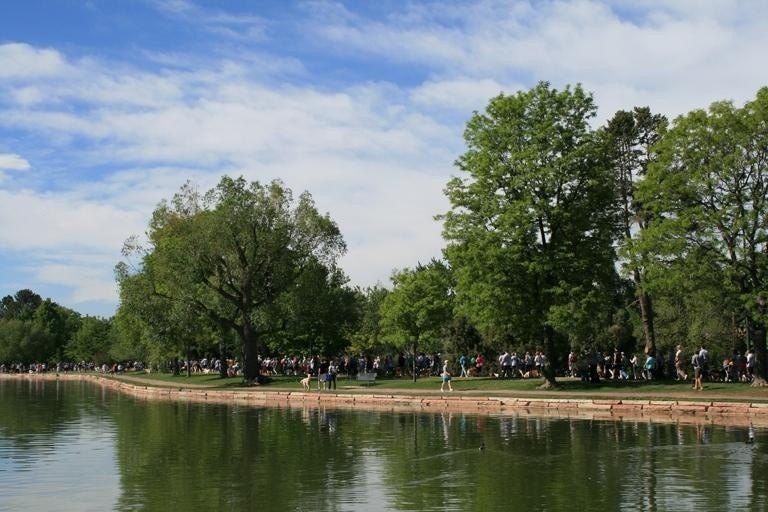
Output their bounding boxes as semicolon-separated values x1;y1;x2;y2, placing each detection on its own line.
300;373;311;391
750;373;768;388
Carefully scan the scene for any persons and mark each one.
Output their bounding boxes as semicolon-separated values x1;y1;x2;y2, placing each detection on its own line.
457;342;768;392
1;362;51;374
178;356;241;374
56;360;143;373
258;354;321;377
317;352;454;391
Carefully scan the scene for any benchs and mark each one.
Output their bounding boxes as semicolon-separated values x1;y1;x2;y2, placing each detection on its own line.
356;372;377;386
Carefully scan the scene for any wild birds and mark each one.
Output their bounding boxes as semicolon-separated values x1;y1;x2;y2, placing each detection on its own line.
478;440;486;451
